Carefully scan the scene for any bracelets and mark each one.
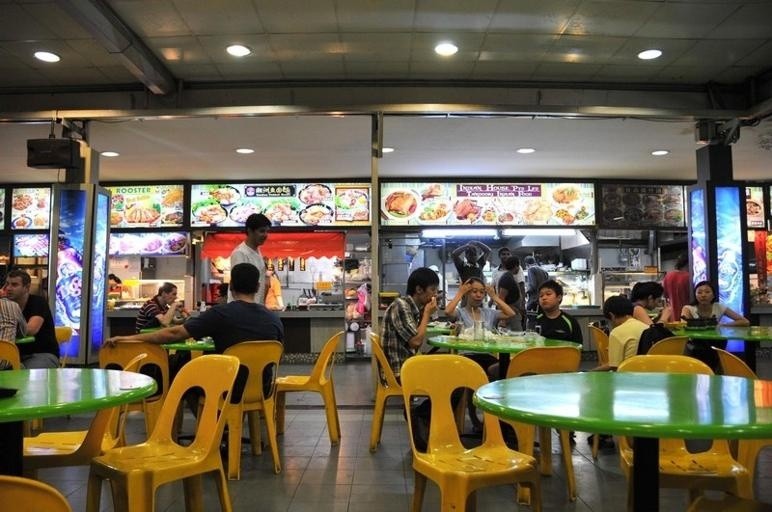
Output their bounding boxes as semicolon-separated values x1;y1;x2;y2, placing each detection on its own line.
653;315;658;322
659;320;665;323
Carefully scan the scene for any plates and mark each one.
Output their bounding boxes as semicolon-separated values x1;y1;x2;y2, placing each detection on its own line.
603;193;684;225
193;183;594;227
109;232;187;256
666;323;687;329
746;201;761;217
571;258;587;271
11;193;185;231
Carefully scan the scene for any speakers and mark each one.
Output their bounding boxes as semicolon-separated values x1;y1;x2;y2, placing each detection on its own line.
27;138;81;169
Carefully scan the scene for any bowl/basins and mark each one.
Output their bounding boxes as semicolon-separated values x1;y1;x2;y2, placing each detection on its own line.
644;265;657;273
601;266;626;272
686;319;718;327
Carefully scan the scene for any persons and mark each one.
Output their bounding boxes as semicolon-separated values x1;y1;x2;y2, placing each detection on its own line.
0;267;29;370
587;295;652;449
381;267;465;452
629;280;674;327
7;269;60;370
445;240;583;445
661;252;690;322
680;280;751;373
107;273;129;293
227;213;272;306
135;261;286;333
100;263;285;450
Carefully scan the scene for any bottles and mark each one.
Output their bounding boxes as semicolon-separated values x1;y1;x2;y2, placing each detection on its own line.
106;299;114;312
286;303;291;311
200;301;206;313
58;234;83;323
474;321;485;344
496;318;541;347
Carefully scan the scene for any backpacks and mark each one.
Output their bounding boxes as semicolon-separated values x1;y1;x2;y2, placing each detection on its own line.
637;323;675;356
